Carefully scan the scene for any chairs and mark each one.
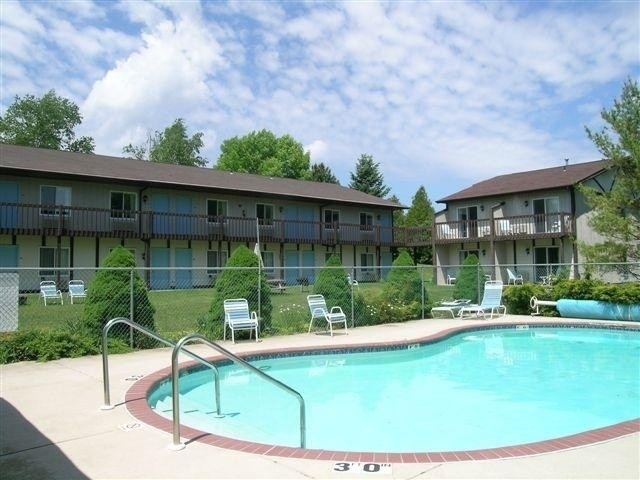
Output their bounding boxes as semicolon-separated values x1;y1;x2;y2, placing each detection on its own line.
38;281;64;306
507;266;523;286
348;277;359;291
307;294;348;337
66;280;88;304
442;225;453;239
446;268;456;285
223;298;259;345
485;271;495;285
460;280;507;321
501;220;512;235
431;298;471;318
462;334;504;358
